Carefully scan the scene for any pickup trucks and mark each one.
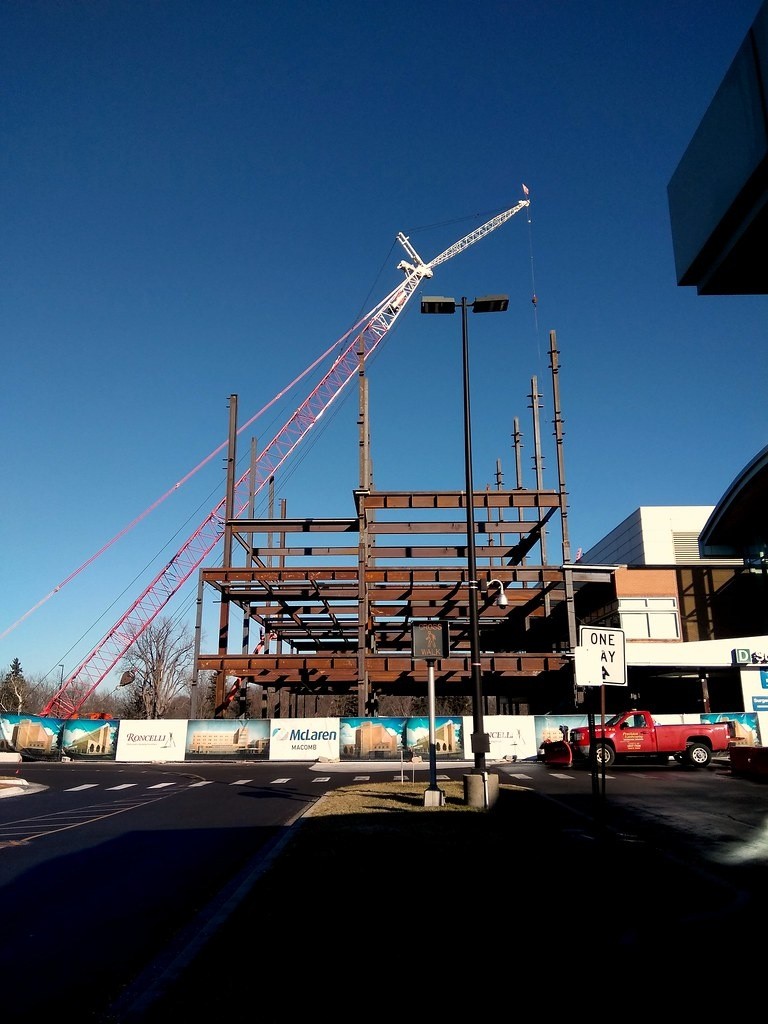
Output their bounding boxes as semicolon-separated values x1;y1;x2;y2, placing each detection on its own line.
570;708;730;768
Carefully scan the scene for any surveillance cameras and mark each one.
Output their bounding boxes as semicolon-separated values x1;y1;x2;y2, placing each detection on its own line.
497;595;508;609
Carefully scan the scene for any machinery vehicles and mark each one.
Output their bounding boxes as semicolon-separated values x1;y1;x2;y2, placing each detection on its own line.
1;185;539;717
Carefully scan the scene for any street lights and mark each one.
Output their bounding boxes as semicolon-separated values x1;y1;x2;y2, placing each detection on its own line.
419;294;511;809
